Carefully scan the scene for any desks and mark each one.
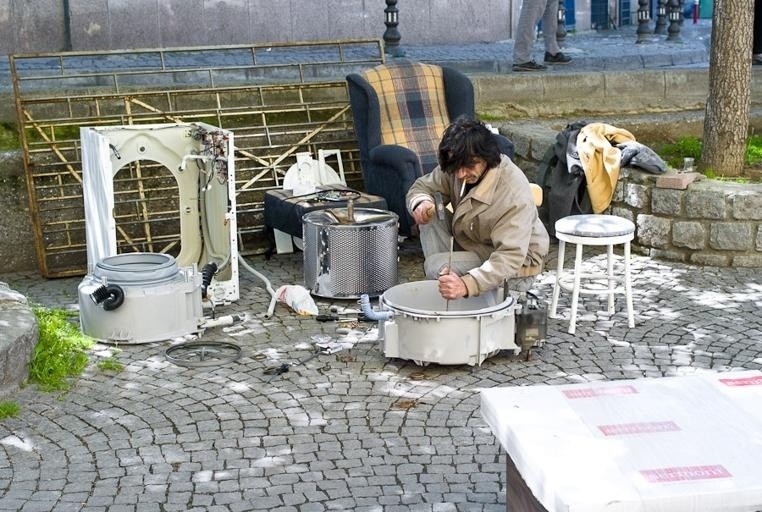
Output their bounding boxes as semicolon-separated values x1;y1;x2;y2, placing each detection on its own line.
480;367;759;509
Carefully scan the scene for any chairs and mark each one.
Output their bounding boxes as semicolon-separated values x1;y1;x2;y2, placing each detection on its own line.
345;60;518;241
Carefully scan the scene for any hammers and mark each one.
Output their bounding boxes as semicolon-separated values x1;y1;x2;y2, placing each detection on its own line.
426;191;445;220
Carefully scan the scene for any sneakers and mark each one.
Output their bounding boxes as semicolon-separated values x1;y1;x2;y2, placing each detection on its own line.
543;51;574;66
512;60;548;73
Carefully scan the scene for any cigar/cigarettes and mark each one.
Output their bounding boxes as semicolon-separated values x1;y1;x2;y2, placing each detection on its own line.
458;178;469;196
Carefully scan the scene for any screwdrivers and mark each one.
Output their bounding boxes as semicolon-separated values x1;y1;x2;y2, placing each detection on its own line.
316;314;358;322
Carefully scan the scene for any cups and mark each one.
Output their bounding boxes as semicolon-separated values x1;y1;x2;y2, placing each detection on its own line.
683;158;695;172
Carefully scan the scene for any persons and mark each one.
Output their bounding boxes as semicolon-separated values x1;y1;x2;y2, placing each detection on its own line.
406;113;550;300
512;0;573;72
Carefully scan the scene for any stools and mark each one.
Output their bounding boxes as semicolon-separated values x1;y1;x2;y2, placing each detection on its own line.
263;186;389;255
549;213;636;335
